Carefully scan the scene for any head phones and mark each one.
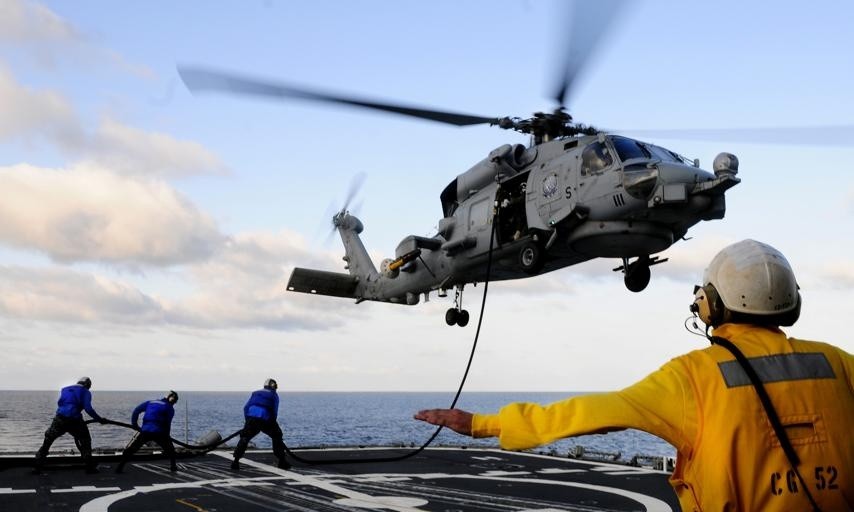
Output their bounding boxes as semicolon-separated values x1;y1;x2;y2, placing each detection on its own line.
169;396;174;403
271;383;277;389
690;284;802;327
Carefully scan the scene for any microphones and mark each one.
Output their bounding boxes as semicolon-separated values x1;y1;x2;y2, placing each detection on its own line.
692;322;706;335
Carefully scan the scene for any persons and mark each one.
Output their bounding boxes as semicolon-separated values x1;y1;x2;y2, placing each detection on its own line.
113;390;181;472
410;238;854;512
30;376;108;475
231;377;292;471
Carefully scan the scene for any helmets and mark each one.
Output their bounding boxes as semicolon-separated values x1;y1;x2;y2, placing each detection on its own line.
166;390;178;404
77;377;92;390
703;239;797;316
264;379;277;390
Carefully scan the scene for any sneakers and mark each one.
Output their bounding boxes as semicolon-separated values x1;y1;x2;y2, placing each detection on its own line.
232;460;240;470
171;462;177;470
117;464;125;473
82;454;97;463
278;458;291;469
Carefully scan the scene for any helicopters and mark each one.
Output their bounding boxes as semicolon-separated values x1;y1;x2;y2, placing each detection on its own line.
176;1;853;329
